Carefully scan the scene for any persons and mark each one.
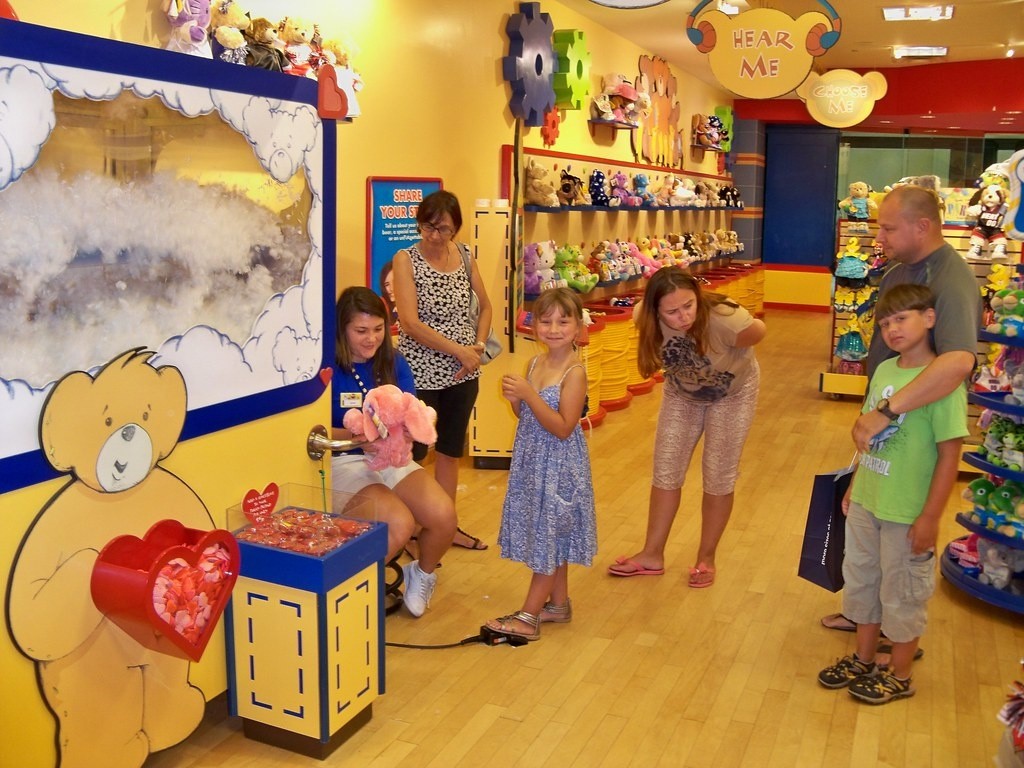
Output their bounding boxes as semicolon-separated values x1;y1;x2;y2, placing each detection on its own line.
822;183;980;668
392;190;493;552
330;287;457;618
378;262;398;326
605;264;766;588
815;290;971;706
484;287;598;641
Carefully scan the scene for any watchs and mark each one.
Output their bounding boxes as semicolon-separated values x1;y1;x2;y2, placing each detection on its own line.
877;399;901;420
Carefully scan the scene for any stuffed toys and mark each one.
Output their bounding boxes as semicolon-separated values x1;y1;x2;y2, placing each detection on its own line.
964;183;1012;261
962;474;1024;539
342;384;438;471
161;0;364;120
976;408;1024;473
591;71;653;126
1002;360;1024;407
977;281;1024;337
523;156;746;209
975;538;1023;589
691;112;731;149
839;181;878;234
523;229;745;327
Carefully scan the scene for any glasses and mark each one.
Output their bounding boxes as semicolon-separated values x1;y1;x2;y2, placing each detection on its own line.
421;222;456;234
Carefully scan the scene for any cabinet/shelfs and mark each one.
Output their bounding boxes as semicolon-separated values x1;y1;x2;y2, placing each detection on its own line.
818;218;1024;401
939;327;1024;614
524;204;746;300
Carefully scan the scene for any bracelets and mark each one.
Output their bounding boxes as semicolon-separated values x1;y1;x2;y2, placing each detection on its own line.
475;341;486;348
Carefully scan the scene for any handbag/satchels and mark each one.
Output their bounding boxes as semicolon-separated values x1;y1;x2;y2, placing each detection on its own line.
798;450;858;593
456;242;502;365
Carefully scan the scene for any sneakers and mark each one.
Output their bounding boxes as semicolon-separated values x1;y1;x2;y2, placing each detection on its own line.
403;559;437;618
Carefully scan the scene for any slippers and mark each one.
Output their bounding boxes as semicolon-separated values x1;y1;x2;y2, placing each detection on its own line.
688;561;717;587
822;613;885;637
853;640;924;665
609;556;665;575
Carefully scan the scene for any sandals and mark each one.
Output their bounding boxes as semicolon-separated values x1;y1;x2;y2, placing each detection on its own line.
452;527;488;549
848;665;915;702
486;610;540;640
818;654;878;687
539;598;571;622
405;536;442;568
